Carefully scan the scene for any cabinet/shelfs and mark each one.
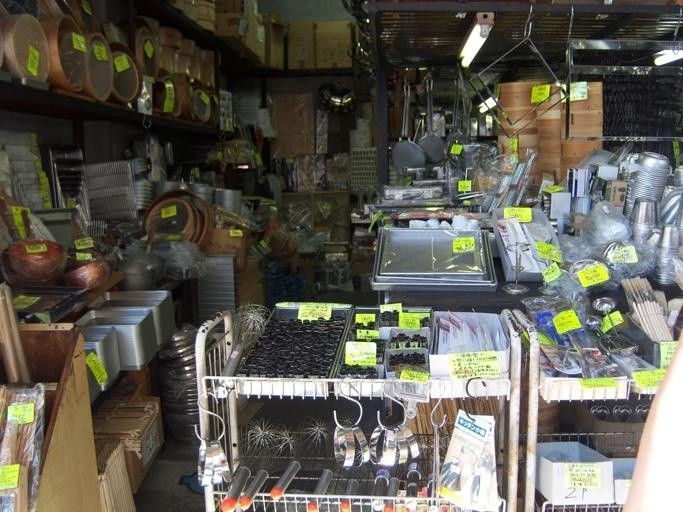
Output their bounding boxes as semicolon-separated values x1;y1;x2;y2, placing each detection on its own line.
0;0;244;140
192;300;522;511
512;307;683;511
284;188;351;248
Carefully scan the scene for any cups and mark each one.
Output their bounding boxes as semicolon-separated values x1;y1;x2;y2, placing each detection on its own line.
629;189;683;286
409;217;476;230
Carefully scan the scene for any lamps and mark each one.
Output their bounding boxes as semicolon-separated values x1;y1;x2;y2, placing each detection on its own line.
476;95;500;115
457;10;497;72
650;49;682;70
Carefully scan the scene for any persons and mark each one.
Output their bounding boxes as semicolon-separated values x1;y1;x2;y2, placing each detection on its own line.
622;306;683;512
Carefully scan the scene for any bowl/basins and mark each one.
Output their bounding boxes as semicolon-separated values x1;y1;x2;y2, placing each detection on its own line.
623;150;671;224
64;261;112;291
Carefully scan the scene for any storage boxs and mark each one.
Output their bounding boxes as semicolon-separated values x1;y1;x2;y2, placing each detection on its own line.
215;9;360;72
